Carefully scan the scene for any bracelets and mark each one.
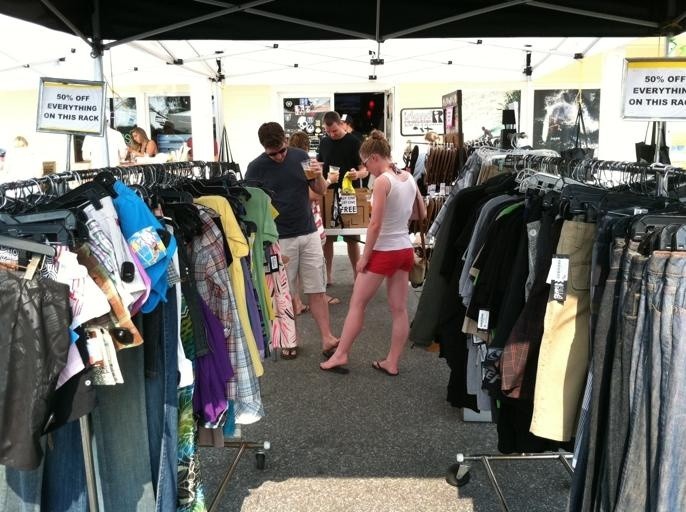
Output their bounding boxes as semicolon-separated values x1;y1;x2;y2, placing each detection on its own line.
327;178;332;185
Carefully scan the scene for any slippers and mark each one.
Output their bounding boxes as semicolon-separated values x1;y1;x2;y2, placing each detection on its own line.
281;346;297;360
327;296;341;305
373;360;399;376
295;304;310;316
320;349;349;374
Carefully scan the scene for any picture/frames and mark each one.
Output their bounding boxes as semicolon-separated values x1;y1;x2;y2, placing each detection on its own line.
399;107;445;136
619;57;686;122
36;76;107;136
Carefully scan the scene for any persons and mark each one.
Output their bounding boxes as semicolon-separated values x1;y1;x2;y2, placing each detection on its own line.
316;111;370;286
82;126;126;169
288;131;341;316
544;88;597;136
319;129;428;375
126;126;159;159
481;127;492;141
340;113;364;143
242;122;344;360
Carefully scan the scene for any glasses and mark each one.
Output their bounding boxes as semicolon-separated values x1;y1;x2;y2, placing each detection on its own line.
268;147;286;156
363;155;371;166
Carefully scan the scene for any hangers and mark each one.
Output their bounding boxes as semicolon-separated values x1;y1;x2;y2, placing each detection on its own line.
0;160;244;283
464;146;686;260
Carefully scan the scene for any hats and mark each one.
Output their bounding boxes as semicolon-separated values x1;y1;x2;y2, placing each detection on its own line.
341;114;353;123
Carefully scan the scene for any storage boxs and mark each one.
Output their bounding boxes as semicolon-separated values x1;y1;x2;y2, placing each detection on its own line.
324;188;369;228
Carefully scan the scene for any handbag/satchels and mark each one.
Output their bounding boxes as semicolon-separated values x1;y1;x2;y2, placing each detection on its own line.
561;148;593;160
409;254;430;288
636;142;671;165
210;161;236;178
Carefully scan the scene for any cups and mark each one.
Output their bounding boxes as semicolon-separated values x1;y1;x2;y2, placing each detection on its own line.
300;158;317;179
328;164;340;183
